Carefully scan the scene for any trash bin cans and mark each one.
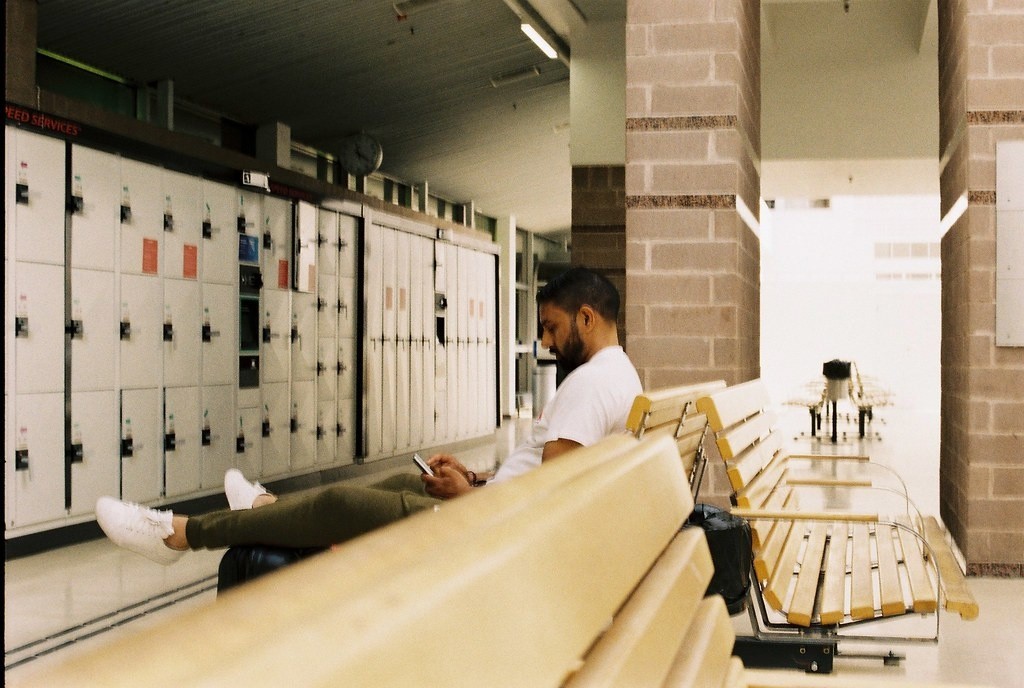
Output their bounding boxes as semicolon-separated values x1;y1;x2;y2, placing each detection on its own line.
822;359;852;400
530;366;557;419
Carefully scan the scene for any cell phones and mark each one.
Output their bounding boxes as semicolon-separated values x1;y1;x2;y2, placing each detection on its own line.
412;454;435;478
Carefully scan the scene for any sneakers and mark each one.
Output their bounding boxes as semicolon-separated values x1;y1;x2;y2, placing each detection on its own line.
223;468;269;510
95;495;187;567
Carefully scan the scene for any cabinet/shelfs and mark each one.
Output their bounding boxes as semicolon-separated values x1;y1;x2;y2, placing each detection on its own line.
4;101;501;560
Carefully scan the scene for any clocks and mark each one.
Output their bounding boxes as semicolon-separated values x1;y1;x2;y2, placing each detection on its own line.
338;133;383;176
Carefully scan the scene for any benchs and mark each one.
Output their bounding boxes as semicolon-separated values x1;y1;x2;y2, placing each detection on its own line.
695;374;978;670
4;433;749;688
625;374;726;499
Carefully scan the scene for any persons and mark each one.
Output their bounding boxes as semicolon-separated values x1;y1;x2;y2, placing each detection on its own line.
95;265;643;566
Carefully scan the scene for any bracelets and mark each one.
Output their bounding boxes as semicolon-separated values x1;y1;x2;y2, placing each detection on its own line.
467;470;477;487
